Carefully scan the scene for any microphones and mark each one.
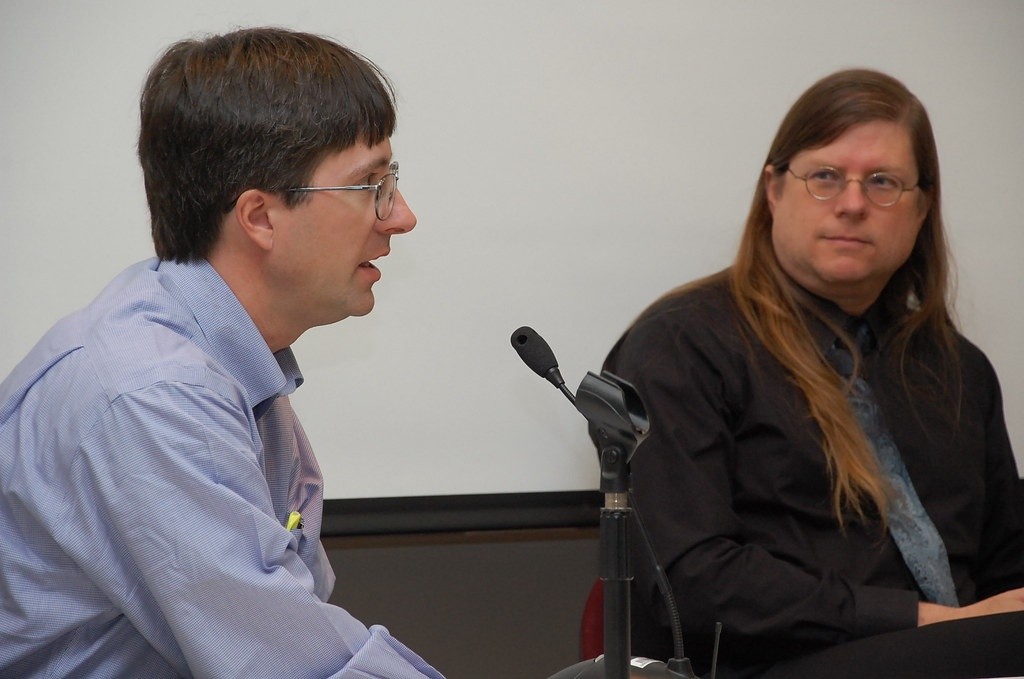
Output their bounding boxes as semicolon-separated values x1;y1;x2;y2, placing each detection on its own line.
509;326;695;679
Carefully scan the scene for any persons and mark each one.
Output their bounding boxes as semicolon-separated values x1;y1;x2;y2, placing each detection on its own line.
589;69;1024;679
0;25;447;679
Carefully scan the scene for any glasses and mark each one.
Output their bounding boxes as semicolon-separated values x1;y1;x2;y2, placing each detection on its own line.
787;166;921;207
223;161;399;221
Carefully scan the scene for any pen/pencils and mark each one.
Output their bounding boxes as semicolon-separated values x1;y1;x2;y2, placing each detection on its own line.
287;511;301;531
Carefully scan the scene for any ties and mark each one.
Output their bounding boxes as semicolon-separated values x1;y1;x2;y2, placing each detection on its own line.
827;329;958;608
